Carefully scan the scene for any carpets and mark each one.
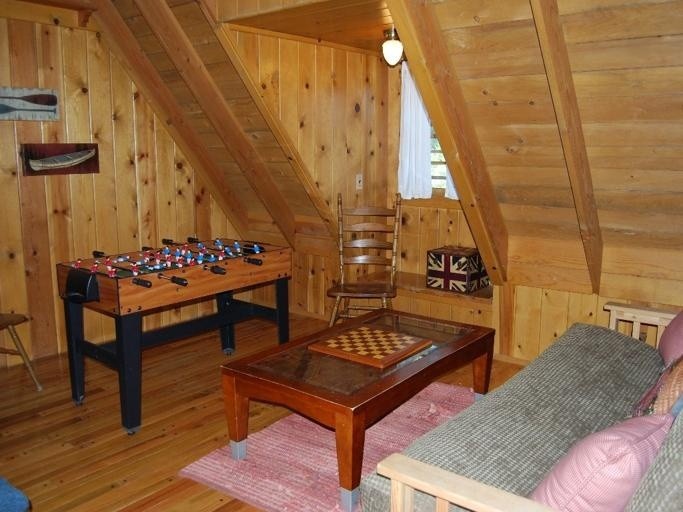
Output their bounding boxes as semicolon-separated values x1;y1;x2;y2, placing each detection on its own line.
179;381;484;511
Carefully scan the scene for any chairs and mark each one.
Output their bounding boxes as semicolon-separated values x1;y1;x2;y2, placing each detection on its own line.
0;314;42;391
328;193;403;327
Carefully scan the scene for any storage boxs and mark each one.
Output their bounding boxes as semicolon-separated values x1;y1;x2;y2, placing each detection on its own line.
426;247;489;294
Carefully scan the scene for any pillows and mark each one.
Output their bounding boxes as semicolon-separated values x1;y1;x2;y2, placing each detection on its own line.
527;413;677;511
649;361;683;413
658;309;683;368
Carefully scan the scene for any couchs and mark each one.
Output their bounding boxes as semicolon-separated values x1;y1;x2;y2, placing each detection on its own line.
361;302;683;512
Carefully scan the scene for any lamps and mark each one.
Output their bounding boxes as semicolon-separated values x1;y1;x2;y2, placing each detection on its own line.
381;26;404;68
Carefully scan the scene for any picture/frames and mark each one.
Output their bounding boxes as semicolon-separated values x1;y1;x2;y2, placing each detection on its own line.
0;88;60;121
22;143;100;176
